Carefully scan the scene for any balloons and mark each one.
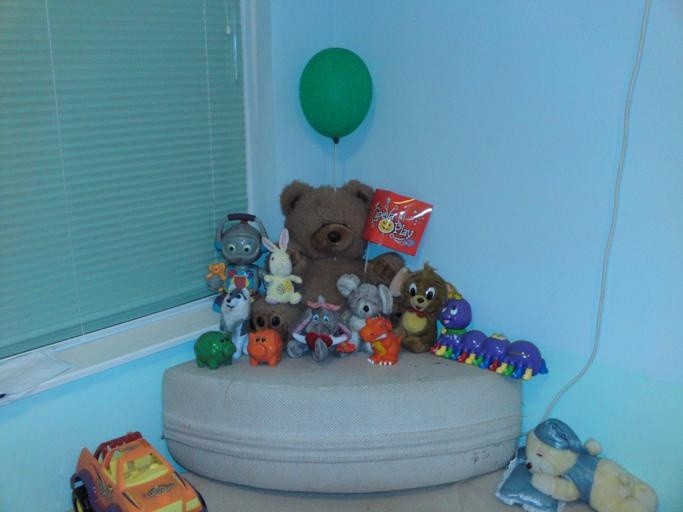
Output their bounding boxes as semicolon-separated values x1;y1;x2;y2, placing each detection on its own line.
300;48;372;144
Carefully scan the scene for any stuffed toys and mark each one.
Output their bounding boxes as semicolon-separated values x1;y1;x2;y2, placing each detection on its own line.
386;260;460;353
191;210;404;366
249;179;406;344
492;418;658;510
